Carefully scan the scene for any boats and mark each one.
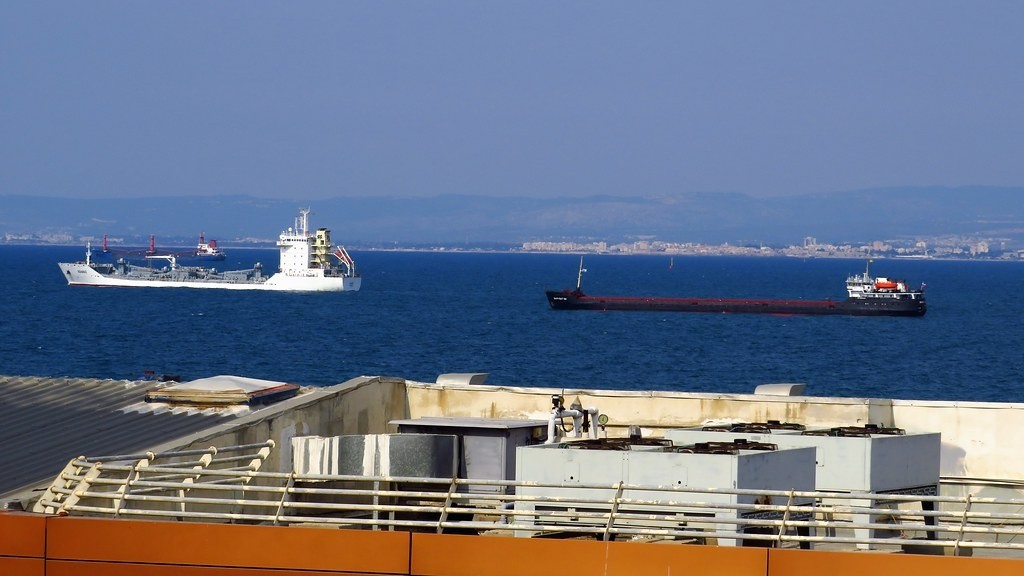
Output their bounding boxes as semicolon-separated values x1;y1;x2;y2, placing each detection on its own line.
544;255;929;317
57;206;363;292
196;233;226;257
96;233;174;259
